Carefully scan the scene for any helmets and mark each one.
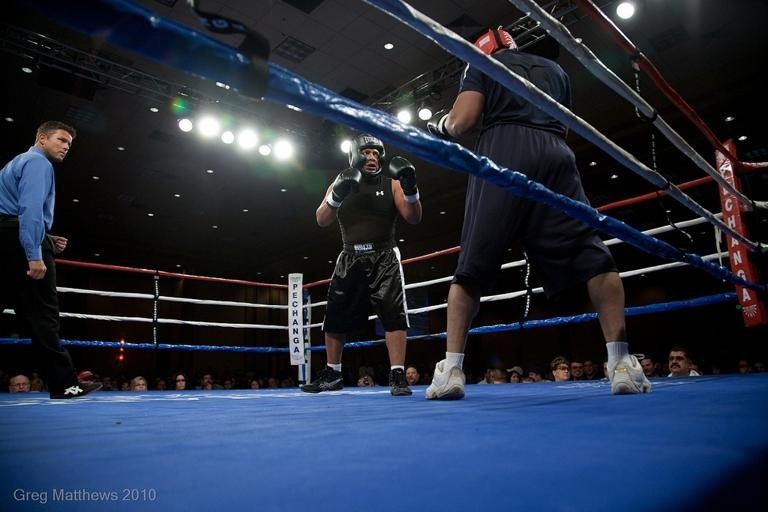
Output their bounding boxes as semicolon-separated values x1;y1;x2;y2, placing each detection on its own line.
349;133;386;168
474;30;518;55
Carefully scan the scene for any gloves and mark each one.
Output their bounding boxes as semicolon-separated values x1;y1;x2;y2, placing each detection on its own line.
389;156;418;196
331;167;362;199
427;108;449;135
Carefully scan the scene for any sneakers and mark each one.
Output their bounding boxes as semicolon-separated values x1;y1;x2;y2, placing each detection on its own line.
49;383;103;399
300;365;343;393
607;354;652;394
389;368;413;396
425;358;466;401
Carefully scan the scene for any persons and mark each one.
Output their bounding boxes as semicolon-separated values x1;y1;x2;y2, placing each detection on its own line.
0;337;768;395
423;32;651;402
0;120;105;400
298;132;424;397
